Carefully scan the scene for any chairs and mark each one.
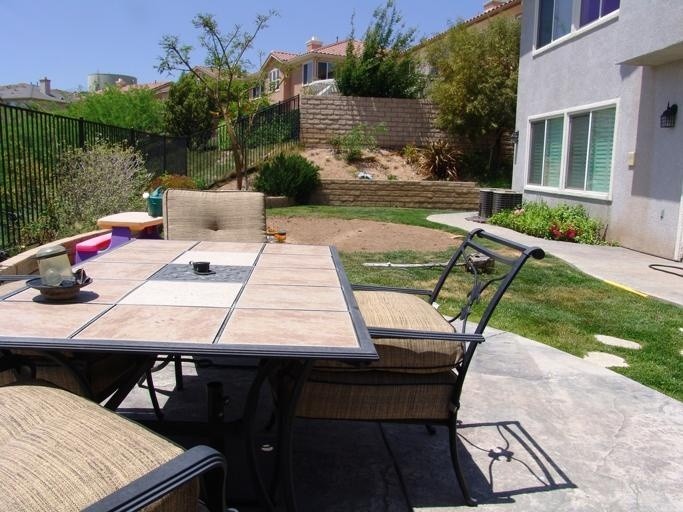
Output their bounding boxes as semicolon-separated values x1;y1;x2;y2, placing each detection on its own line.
0;383;227;512
163;189;268;243
215;229;542;505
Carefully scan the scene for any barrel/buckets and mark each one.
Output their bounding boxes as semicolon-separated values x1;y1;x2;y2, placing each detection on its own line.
478;189;522;220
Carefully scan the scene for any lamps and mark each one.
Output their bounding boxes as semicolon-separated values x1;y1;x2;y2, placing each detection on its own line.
659;100;677;127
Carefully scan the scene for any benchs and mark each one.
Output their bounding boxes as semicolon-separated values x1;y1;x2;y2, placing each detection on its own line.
75;229;113;264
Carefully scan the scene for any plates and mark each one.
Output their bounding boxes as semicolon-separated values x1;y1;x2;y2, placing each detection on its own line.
24;274;94;299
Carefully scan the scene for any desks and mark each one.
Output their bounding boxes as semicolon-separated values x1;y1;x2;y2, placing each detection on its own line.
1;238;379;424
96;210;163;229
